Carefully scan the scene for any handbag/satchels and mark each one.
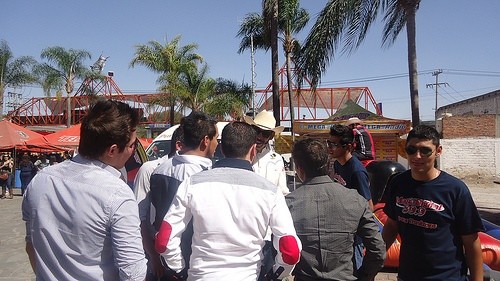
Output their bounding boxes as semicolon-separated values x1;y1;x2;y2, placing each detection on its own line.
0;171;8;180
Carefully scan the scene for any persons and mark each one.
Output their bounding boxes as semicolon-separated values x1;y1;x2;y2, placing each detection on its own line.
21;100;485;281
0;150;75;200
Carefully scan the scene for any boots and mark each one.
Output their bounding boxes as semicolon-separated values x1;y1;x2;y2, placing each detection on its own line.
9;187;13;198
1;187;6;198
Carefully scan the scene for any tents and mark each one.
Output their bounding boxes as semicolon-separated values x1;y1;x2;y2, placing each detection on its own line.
0;119;157;189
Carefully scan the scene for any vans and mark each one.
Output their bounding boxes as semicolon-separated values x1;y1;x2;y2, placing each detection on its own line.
143;120;230;168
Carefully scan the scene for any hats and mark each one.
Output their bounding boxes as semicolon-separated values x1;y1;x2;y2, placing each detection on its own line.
243;109;284;135
345;117;364;126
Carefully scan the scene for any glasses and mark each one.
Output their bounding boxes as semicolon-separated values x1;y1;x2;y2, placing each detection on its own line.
125;136;139;149
254;127;272;137
327;139;347;147
404;144;436;156
